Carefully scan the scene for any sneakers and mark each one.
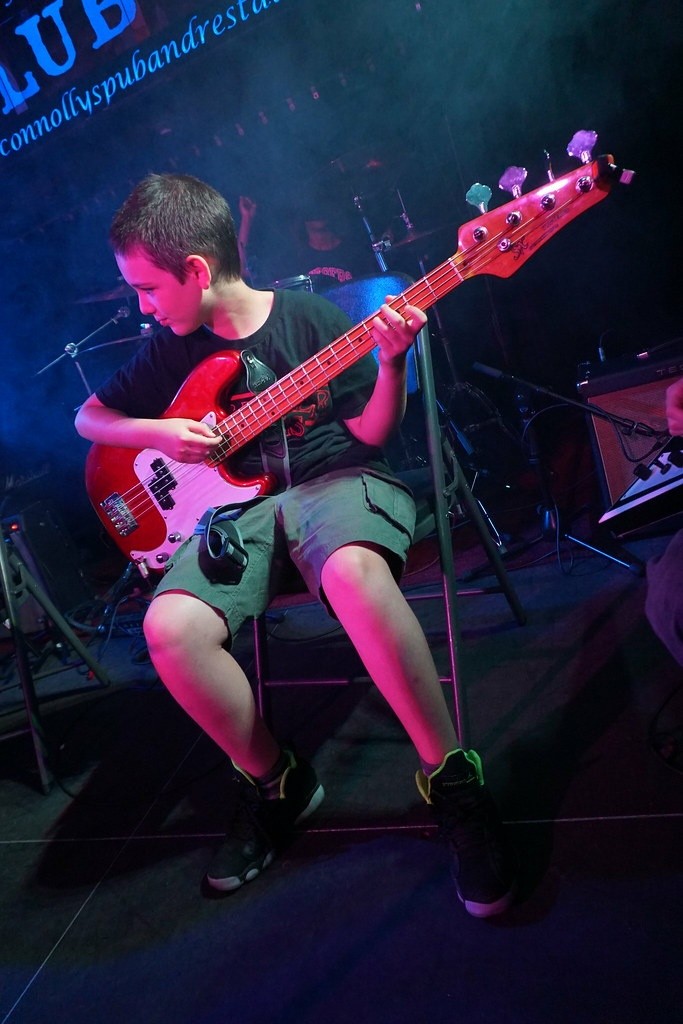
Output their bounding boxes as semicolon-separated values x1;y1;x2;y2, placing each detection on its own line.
205;748;326;893
416;748;514;918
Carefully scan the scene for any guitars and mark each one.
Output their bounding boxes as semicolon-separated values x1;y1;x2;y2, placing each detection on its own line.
81;127;639;573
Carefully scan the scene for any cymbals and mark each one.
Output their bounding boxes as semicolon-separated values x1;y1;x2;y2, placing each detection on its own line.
297;137;414;214
381;222;448;255
69;283;137;306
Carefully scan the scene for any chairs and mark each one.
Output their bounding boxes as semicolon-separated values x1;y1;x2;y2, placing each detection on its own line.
251;271;525;760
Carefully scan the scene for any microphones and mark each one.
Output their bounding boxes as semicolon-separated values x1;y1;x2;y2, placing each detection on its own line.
652;429;670;451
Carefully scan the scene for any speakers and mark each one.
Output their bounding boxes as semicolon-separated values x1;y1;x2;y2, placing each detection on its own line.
0;498;90;639
577;344;683;538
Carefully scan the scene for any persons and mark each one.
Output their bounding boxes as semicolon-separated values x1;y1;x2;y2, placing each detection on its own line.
233;193;374;295
644;375;683;672
73;169;519;917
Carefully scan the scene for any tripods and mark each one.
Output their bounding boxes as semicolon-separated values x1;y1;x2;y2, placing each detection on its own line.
463;362;652;582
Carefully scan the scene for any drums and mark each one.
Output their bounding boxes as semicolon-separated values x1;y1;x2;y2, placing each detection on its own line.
258;275;344;295
